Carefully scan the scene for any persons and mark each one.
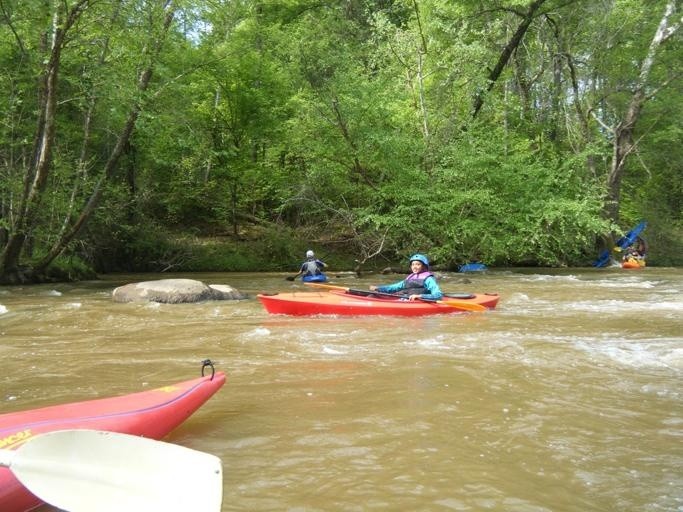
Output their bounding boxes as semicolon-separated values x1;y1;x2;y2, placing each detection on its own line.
369;254;442;302
297;250;329;278
622;246;638;261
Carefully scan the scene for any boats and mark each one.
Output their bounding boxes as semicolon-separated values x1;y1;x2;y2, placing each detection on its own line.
597;222;646;267
301;272;326;282
257;291;501;318
0;371;226;511
459;262;487;271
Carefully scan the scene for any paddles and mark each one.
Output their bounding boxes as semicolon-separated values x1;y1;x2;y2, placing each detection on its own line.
285;254;329;282
637;260;645;267
614;246;628;252
0;427;224;511
305;281;487;312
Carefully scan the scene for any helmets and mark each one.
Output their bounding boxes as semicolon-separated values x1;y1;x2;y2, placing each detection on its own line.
410;254;430;270
306;251;314;260
